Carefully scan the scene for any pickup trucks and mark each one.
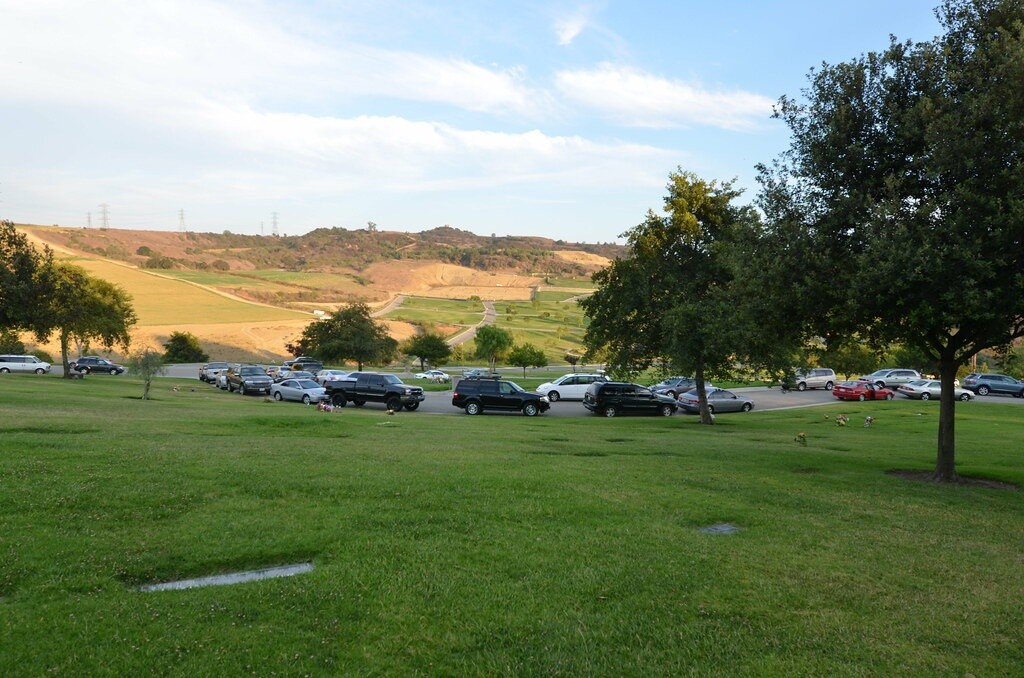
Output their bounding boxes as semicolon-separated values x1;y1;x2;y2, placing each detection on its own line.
322;372;425;413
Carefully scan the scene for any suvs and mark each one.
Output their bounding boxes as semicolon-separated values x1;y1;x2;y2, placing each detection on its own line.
649;375;713;399
0;354;52;375
962;373;1024;399
581;380;679;417
860;368;921;389
781;368;837;391
462;368;495;379
452;376;550;416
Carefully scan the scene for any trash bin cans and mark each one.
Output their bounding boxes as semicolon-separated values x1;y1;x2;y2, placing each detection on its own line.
452;375;462;391
319;395;332;410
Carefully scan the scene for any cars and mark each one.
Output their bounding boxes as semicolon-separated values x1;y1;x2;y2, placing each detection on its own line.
270;378;331;406
67;356;124;376
676;386;756;416
414;369;449;382
535;369;613;403
198;356;378;395
833;379;896;401
897;379;976;403
